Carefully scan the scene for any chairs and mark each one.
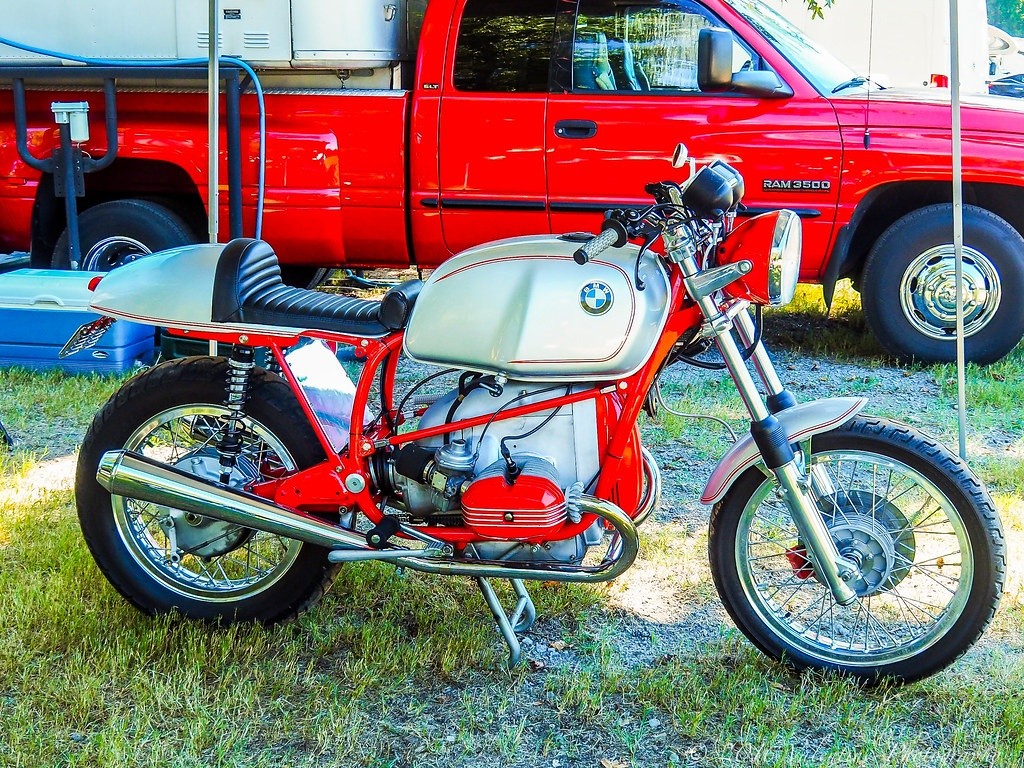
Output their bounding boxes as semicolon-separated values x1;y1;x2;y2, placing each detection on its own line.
555;30;616;90
607;38;651;91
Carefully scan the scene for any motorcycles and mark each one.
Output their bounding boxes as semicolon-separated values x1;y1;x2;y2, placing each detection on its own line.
62;139;1008;688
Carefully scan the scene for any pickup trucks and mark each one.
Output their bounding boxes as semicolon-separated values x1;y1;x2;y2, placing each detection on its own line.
0;0;1024;371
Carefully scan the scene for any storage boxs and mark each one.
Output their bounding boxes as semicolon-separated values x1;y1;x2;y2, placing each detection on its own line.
0;269;161;385
